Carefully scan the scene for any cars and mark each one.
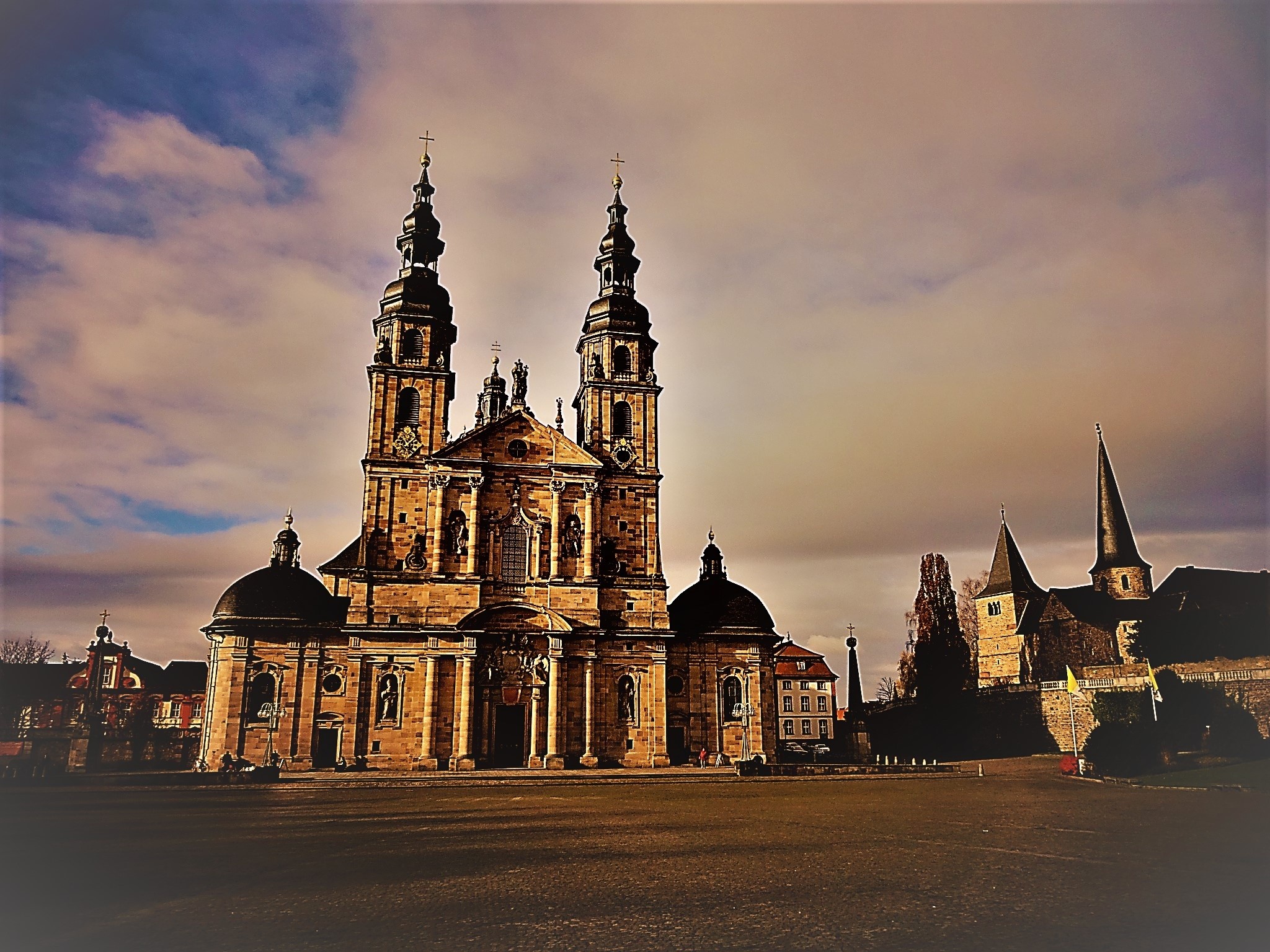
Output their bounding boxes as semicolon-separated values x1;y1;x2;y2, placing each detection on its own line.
787;741;825;756
815;744;830;752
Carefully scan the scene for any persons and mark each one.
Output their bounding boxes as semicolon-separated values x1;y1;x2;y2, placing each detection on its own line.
518;650;548;684
222;751;232;767
271;750;281;768
588;352;604;379
494;634;533;655
619;680;635;719
752;753;764;775
479;654;496;684
511;359;528;404
374;336;394;365
698;748;707;769
564;519;581;557
451;516;469;555
381;679;397;721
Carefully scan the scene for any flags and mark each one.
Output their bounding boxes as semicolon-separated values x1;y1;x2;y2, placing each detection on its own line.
1146;658;1163;702
1066;665;1090;701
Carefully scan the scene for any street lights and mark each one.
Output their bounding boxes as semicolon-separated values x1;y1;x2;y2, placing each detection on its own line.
731;702;756;761
258;701;287;768
782;722;787;753
